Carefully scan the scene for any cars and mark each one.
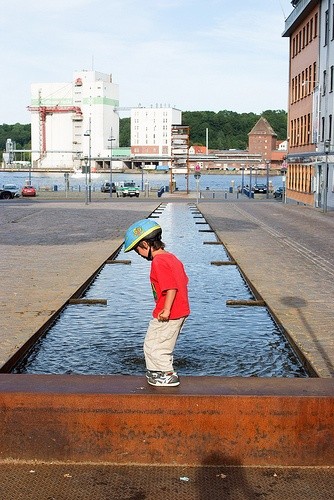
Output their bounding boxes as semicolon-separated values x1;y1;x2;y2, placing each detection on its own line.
273;186;285;199
101;183;116;193
252;182;267;194
0;183;20;200
21;185;37;197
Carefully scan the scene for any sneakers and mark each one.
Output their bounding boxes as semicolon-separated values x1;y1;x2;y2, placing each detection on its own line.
146;371;181;386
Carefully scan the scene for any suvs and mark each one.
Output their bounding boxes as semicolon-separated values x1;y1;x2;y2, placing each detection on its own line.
115;180;140;198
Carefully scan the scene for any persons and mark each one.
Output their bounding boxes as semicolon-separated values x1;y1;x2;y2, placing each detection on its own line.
124;219;190;387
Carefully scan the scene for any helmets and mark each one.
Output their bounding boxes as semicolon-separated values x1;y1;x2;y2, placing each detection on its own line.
124;219;162;253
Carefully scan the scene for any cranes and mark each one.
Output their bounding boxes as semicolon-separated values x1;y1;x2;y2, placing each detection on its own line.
26;77;83;157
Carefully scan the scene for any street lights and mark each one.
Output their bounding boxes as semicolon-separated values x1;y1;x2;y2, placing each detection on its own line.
27;163;32;185
323;139;330;213
265;159;270;199
249;161;253;199
83;117;93;203
83;156;89;206
108;126;116;198
282;154;288;202
241;164;245;195
140;165;145;192
